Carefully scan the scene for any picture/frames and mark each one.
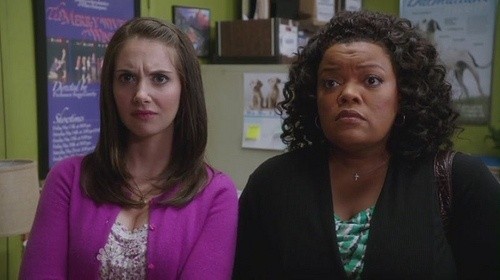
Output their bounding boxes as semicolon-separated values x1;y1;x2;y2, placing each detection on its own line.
172;5;211;59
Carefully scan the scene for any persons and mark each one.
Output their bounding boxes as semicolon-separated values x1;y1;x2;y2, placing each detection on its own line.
17;16;239;280
230;10;500;280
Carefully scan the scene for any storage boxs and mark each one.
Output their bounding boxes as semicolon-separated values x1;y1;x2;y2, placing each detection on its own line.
216;18;298;65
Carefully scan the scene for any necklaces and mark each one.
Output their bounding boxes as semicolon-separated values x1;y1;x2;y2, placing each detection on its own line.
351;167;362;183
132;178;152;209
